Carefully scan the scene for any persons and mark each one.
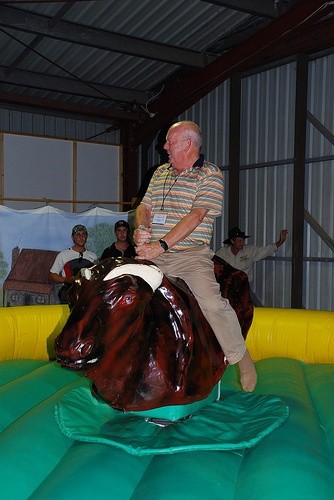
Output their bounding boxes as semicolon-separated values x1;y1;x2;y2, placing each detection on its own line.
100;220;137;261
134;121;259;392
215;226;288;307
50;224;97;304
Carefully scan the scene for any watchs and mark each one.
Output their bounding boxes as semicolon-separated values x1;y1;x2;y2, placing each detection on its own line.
157;239;169;252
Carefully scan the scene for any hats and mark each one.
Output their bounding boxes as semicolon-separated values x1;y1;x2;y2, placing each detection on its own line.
115;220;130;233
72;225;87;236
222;228;251;245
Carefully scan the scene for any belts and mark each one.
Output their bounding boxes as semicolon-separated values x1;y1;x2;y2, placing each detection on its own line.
163;244;209;253
